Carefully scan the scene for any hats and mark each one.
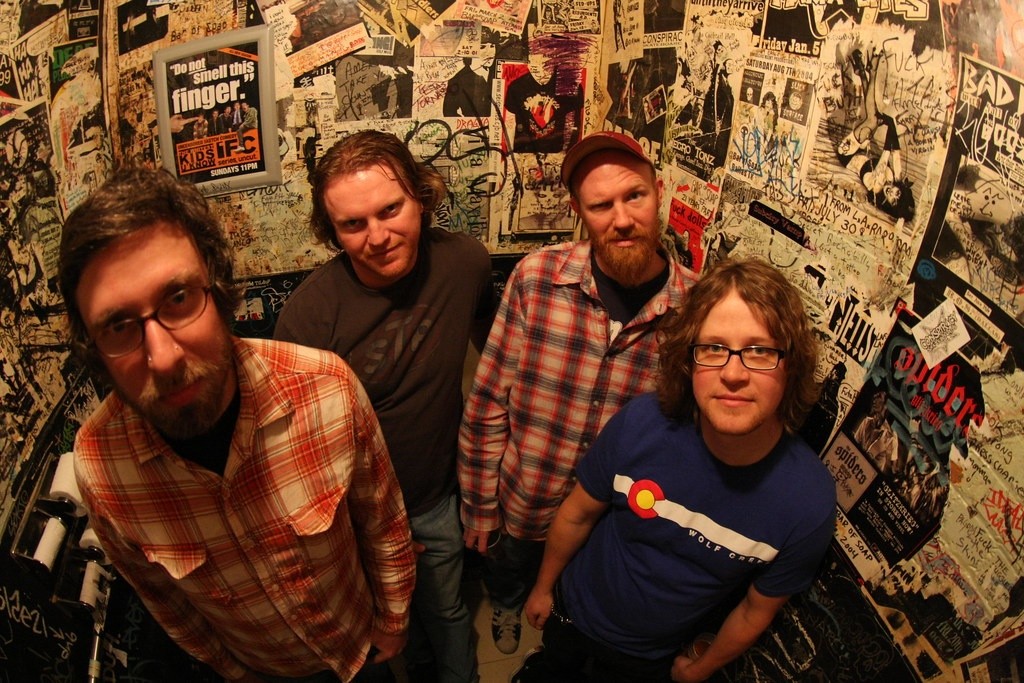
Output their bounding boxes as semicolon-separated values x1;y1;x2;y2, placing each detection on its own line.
560;130;653;188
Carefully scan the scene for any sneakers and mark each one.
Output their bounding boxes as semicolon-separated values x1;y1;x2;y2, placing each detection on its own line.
490;603;525;656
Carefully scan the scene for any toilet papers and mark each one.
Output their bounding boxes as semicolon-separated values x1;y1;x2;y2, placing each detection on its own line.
79;519;113;565
78;562;113;608
47;452;88;517
32;518;67;572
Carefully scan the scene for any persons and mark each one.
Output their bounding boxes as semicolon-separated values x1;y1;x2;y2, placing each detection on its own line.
510;258;836;683
0;0;1022;683
460;131;702;653
273;131;500;683
58;167;417;683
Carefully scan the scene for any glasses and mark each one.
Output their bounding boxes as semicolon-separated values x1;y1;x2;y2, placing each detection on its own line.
689;343;785;371
84;263;219;359
